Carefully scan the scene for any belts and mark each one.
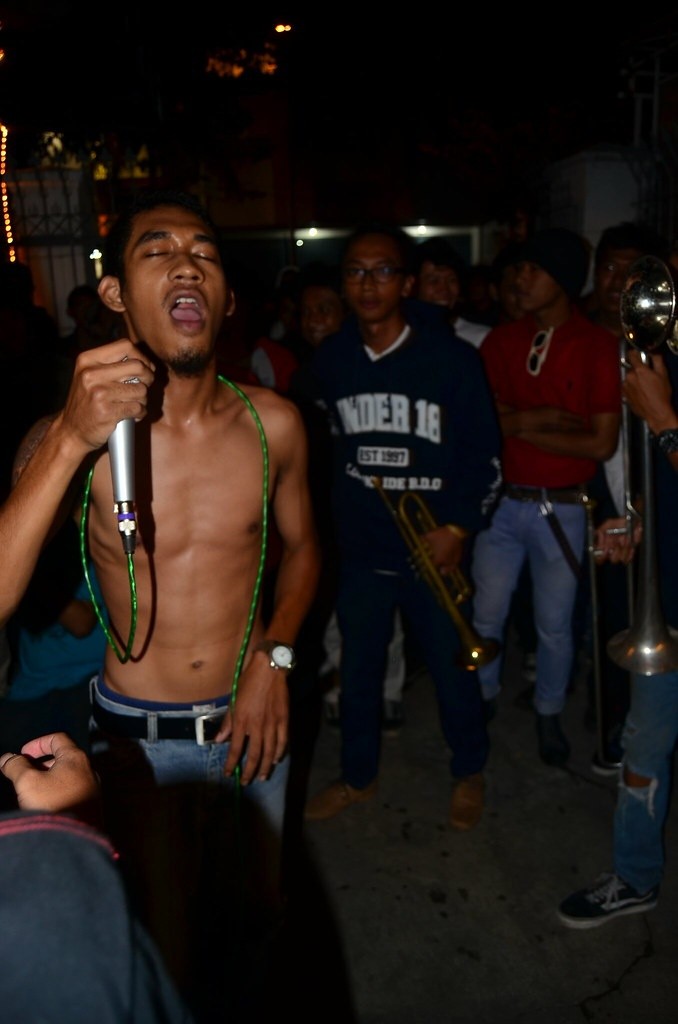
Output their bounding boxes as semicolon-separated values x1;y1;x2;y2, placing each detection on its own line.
500;484;587;504
87;691;250;747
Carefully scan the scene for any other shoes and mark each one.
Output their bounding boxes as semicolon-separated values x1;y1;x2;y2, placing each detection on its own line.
382;697;406;739
590;722;626;776
321;699;344;735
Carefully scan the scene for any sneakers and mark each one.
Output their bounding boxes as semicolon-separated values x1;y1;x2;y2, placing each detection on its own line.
556;870;663;930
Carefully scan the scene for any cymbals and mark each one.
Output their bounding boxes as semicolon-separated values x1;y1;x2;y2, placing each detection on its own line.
620;253;677;348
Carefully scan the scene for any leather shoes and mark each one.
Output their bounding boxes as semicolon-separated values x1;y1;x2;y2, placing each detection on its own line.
303;778;378;821
450;772;484;832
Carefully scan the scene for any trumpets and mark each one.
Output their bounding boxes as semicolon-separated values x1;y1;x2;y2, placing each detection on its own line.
368;475;504;670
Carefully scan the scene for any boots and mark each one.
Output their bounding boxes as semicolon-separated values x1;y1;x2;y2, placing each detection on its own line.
535;712;573;771
482;683;500;720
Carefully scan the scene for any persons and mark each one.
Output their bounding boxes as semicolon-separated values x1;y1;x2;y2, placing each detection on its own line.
0;260;127;817
216;213;678;931
0;189;323;1024
0;731;195;1024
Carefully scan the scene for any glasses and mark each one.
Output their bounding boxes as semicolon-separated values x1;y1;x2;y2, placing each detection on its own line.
341;263;408;283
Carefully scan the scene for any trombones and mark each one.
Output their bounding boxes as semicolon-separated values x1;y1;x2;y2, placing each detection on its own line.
571;339;677;778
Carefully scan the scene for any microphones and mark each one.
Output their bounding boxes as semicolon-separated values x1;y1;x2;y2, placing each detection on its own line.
105;377;139;553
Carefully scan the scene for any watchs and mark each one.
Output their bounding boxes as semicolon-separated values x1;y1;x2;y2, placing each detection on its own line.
252;640;297;671
657;425;678;454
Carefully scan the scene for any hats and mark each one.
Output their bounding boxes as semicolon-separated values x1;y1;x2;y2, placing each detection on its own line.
508;226;589;298
